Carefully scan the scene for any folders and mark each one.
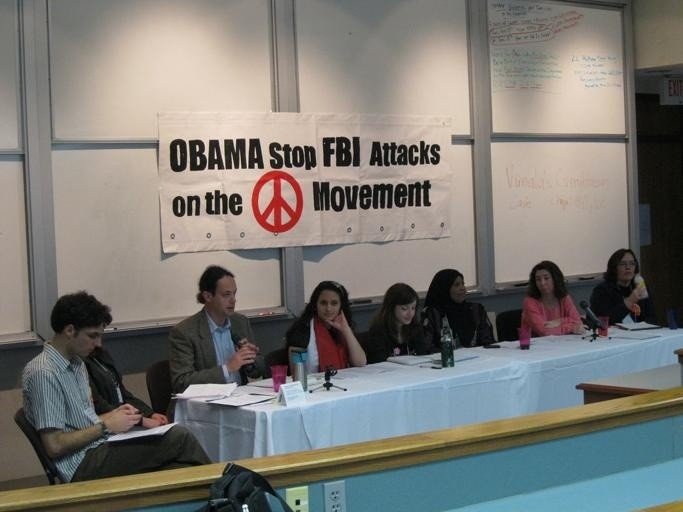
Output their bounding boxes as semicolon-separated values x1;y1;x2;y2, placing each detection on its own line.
203;392;277;406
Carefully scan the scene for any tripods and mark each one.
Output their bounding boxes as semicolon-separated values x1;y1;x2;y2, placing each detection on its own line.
580;317;612;342
308;366;348;394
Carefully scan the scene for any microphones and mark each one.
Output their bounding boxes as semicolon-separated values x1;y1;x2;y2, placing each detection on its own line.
231;332;259;370
578;299;605;330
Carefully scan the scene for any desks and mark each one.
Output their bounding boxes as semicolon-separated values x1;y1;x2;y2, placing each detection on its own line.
576;363;682;404
173;324;683;463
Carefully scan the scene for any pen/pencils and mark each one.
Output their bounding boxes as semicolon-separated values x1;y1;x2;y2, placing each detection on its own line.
419;365;442;370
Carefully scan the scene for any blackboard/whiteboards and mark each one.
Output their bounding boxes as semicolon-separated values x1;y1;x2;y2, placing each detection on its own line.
303;139;478;304
490;136;629;283
49;142;284;324
292;0;472;136
44;0;276;139
0;154;34;335
0;0;24;151
485;0;626;134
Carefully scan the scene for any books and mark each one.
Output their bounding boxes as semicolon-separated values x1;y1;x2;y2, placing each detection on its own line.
171;383;238;400
387;353;433;365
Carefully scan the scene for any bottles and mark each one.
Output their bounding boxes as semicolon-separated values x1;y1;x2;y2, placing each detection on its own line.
635;273;648;297
440;326;454;367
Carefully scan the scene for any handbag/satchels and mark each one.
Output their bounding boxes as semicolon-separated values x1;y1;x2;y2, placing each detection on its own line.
206;462;294;511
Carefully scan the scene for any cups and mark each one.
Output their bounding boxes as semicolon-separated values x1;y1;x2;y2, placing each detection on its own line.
518;328;531;349
271;365;288;393
598;316;608;337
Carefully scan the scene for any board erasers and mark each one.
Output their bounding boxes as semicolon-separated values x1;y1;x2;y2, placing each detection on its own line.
514;282;529;288
354;298;372;304
578;276;595;280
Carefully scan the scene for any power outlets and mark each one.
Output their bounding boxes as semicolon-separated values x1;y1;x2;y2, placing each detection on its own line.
323;480;346;512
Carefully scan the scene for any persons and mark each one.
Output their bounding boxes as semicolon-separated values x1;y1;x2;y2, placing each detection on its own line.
422;269;496;354
519;261;589;337
74;340;167;432
167;266;266;393
285;280;367;372
587;249;659;327
364;283;431;362
20;291;212;481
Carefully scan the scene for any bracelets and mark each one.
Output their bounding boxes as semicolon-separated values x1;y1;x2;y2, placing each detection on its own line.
100;420;112;440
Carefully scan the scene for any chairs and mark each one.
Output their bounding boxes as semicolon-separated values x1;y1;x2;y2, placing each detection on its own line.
146;360;177;422
496;307;523;343
263;348;292;378
14;407;61;486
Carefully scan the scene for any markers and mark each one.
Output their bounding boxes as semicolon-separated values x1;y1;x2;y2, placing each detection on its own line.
466;289;478;293
104;327;119;331
258;311;276;316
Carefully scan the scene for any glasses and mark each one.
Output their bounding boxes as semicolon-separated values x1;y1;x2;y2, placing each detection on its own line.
618;262;637;267
